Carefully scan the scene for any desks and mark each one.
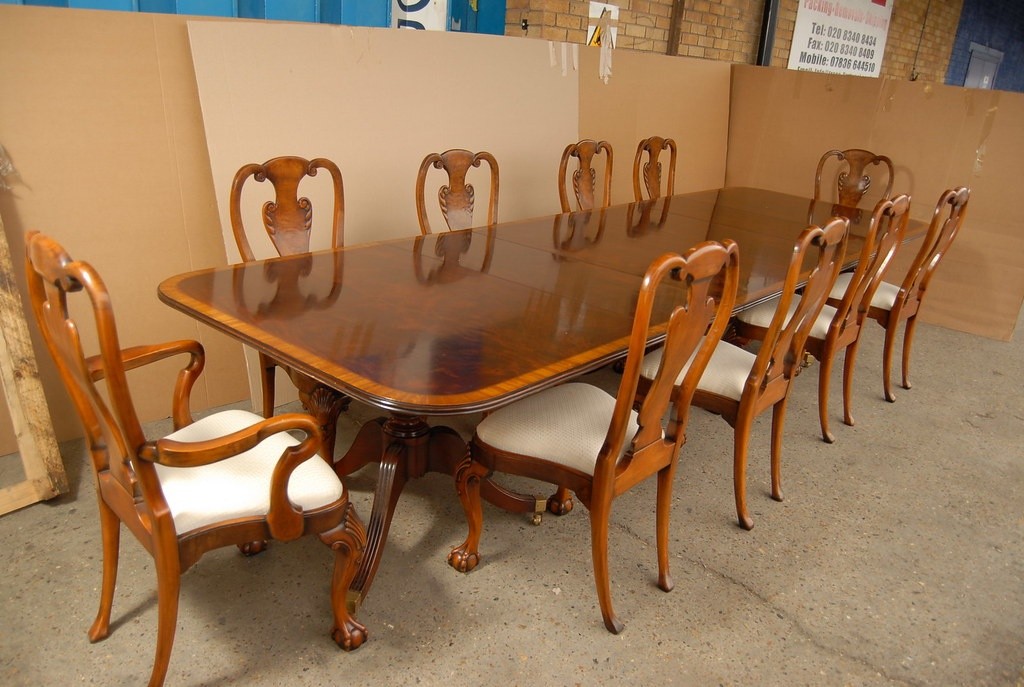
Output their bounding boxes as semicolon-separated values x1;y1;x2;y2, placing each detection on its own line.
157;187;931;617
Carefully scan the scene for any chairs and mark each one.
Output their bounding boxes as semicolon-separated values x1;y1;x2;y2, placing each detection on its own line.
229;155;345;419
633;136;678;202
637;216;850;531
447;239;739;634
723;193;911;444
814;149;894;208
23;229;368;687
415;149;499;236
558;139;613;214
822;186;971;403
